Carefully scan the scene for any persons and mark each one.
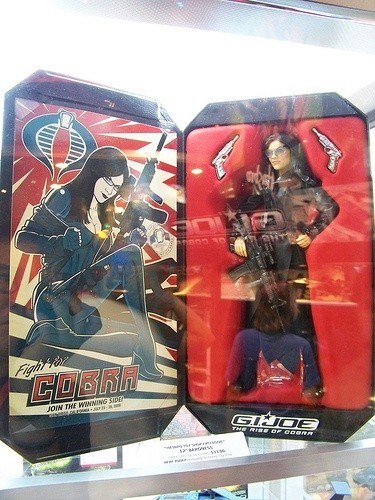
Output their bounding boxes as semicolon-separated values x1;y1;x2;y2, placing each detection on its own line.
232;130;326;400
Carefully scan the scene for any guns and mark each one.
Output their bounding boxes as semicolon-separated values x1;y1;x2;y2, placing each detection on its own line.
312;128;342;173
212;134;239;180
228;214;287;333
